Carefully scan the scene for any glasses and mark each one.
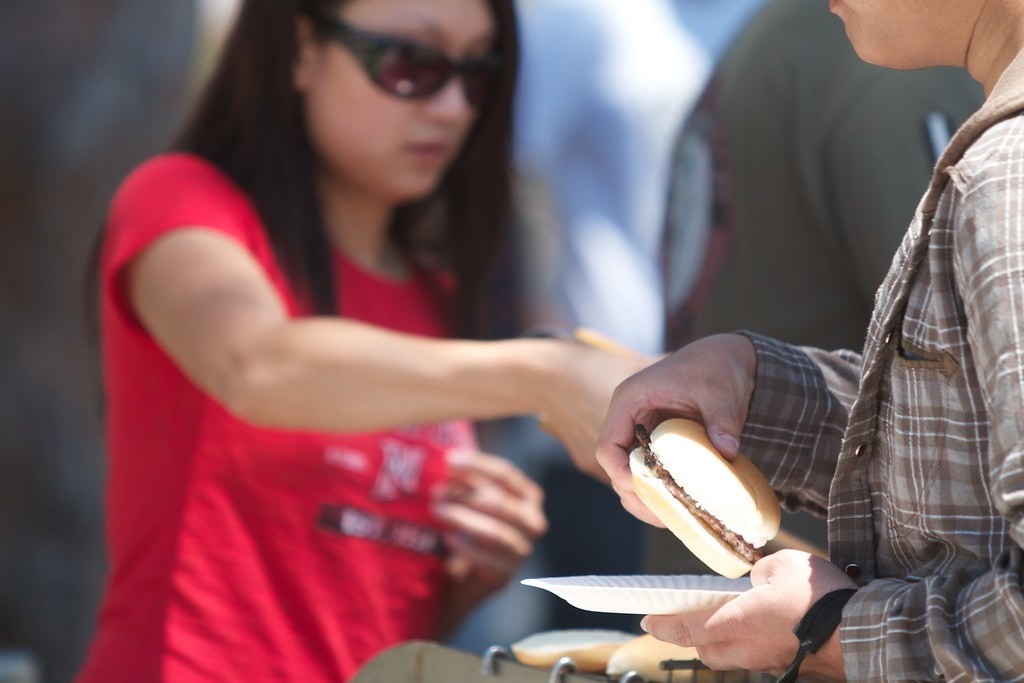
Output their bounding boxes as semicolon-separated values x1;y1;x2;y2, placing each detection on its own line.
311;6;505;112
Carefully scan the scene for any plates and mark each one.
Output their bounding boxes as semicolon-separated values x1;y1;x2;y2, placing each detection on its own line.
522;576;748;617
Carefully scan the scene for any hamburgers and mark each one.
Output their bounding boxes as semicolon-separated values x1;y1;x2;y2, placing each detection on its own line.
628;417;780;578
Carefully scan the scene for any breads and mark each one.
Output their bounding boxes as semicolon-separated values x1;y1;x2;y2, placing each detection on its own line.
607;632;740;682
512;628;637;670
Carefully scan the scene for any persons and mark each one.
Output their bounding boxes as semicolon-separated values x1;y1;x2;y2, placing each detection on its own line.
0;0;1024;683
71;0;652;683
